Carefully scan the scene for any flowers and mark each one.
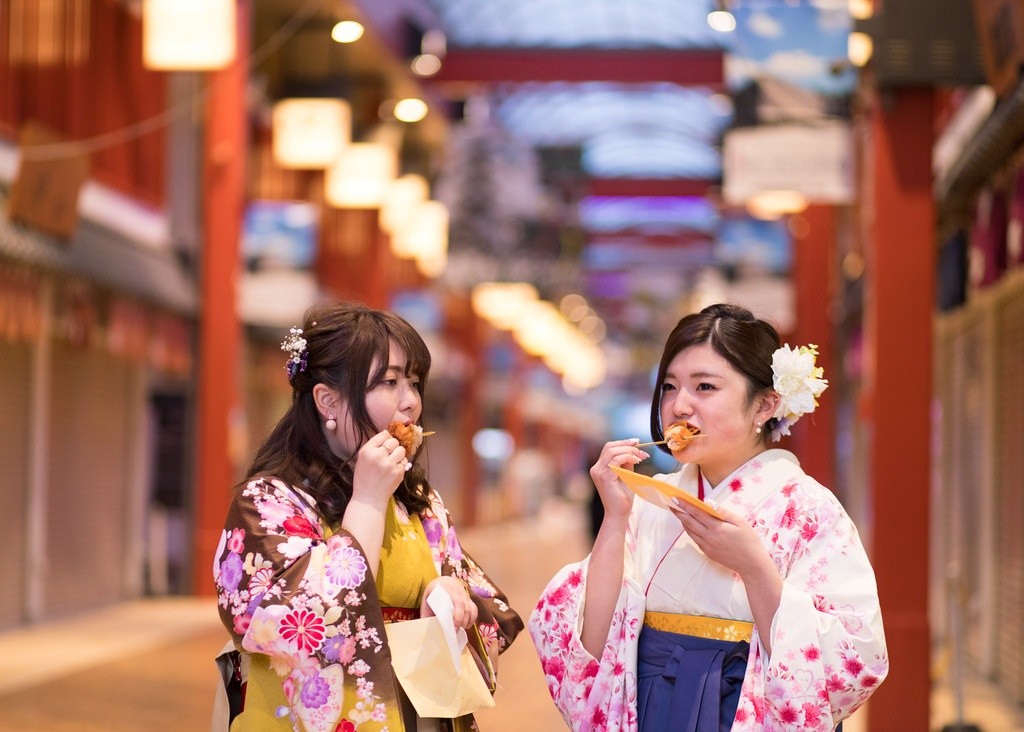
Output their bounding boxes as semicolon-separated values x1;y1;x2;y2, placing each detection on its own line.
281;327;308;381
773;343;831;444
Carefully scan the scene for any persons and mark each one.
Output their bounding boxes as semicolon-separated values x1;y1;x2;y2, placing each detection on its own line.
528;302;891;732
212;300;525;732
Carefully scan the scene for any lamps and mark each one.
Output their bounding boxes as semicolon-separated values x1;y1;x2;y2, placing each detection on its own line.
719;113;857;221
269;13;462;281
